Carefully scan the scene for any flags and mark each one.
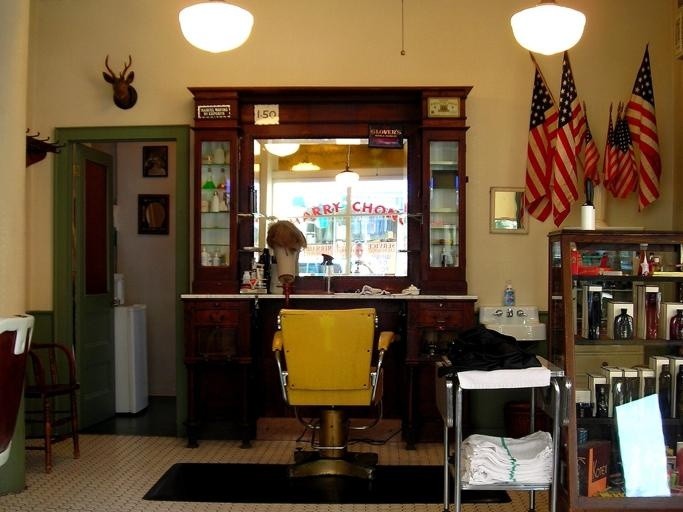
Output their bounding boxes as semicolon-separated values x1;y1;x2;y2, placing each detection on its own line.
584;102;639;199
522;67;559;223
552;52;587;227
624;43;662;212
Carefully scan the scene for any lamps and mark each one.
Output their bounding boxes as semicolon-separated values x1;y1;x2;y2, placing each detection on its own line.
178;1;255;54
509;1;587;57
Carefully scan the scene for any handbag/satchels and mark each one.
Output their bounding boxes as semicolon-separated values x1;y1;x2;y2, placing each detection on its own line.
447;325;542;371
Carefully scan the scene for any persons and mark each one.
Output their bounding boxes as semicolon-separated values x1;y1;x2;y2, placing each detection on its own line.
267;220;307;284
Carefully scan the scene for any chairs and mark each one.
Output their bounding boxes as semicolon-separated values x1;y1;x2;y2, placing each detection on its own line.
24;342;82;472
272;307;396;479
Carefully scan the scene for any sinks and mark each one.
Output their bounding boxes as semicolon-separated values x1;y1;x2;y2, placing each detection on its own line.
478;305;546;341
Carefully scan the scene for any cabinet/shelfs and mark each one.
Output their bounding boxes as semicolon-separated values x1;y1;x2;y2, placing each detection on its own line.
181;87;472;295
435;356;564;512
185;299;478;448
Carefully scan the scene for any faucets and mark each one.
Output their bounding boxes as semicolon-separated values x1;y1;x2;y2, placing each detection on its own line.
506;309;513;317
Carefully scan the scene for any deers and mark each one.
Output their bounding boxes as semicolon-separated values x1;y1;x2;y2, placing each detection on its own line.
103;54;137;109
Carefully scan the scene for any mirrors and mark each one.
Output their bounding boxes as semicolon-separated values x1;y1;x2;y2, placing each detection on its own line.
490;187;529;234
242;124;421;290
562;233;683;512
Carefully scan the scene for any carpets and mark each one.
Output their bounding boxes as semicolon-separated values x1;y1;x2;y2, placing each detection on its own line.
142;462;512;504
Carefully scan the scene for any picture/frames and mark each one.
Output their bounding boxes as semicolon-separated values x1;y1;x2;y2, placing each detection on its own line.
143;145;168;177
138;194;170;234
